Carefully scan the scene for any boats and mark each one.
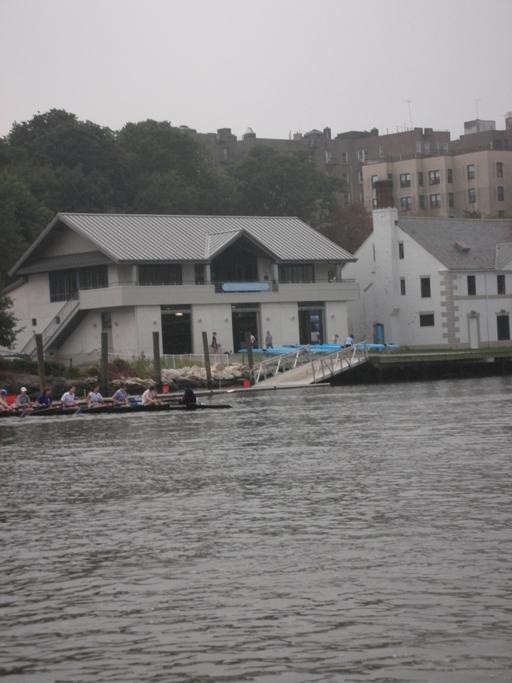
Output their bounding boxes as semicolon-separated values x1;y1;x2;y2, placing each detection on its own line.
0;400;234;417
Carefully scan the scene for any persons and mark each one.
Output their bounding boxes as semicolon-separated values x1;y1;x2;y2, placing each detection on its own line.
249;332;256;349
332;334;339;344
0;382;128;417
210;332;219;354
141;386;168;407
345;333;355;347
264;331;273;349
177;386;196;406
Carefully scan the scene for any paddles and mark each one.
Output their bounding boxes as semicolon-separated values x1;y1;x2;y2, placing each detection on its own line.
72;403;82;414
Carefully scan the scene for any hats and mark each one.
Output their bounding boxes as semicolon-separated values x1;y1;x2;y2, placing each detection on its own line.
0;390;6;393
21;387;27;392
